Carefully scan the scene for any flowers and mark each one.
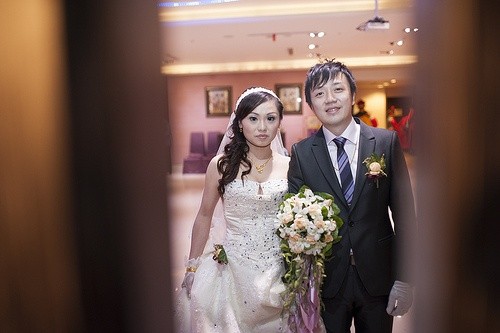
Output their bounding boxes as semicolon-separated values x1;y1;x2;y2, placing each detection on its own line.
213;244;229;266
274;184;344;263
361;153;388;188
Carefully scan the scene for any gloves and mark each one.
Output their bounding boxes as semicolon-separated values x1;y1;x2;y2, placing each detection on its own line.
386;280;412;317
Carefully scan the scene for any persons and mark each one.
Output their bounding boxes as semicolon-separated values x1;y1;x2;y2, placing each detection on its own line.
174;87;323;333
287;58;418;333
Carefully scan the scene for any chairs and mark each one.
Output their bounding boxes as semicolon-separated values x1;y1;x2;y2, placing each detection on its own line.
200;131;221;173
183;131;206;174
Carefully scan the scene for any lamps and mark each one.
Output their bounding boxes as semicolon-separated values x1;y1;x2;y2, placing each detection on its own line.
366;0;391;30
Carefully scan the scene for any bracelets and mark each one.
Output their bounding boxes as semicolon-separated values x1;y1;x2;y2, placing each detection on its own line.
186;266;197;272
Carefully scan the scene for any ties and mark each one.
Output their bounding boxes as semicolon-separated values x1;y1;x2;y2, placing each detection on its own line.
332;137;354;207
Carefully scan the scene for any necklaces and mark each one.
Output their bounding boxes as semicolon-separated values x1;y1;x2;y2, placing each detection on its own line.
246;154;271;174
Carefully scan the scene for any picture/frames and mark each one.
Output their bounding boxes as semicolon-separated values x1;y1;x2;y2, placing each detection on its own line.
275;82;303;115
205;84;233;116
